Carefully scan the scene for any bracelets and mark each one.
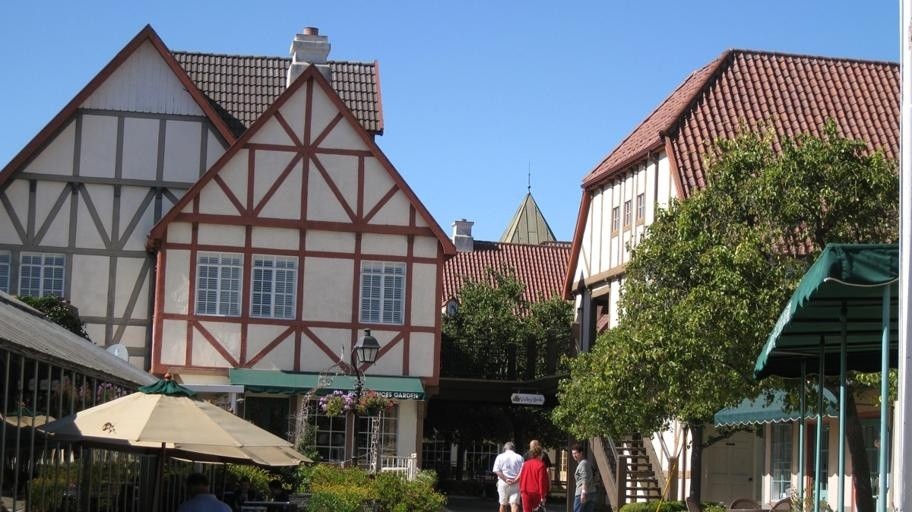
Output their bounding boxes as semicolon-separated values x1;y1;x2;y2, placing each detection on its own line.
505;478;510;482
581;490;587;494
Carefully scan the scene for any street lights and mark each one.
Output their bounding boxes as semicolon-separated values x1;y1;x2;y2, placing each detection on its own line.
350;327;382;467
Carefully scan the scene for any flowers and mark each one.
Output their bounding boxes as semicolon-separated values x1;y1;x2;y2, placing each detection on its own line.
317;390;399;417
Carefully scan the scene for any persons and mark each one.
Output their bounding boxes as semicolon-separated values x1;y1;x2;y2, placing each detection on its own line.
570;443;597;511
518;445;550;511
269;480;294;502
232;477;264;511
177;473;233;512
523;440;552;512
491;441;525;512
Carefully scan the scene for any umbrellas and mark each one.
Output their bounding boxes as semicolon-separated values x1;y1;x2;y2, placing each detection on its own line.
35;372;316;511
0;401;57;429
